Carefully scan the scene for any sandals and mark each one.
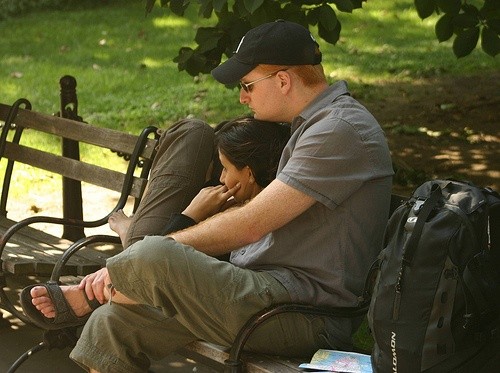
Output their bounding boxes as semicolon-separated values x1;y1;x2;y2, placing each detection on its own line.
20;281;105;331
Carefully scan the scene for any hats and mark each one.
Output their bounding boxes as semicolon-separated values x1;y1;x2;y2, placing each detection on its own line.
211;18;323;89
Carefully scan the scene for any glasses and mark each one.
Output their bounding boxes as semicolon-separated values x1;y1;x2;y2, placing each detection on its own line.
239;64;292;93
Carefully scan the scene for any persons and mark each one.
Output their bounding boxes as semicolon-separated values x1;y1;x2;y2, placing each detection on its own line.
20;20;394;373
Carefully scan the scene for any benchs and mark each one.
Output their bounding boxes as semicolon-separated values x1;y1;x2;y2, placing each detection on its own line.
0;98;412;373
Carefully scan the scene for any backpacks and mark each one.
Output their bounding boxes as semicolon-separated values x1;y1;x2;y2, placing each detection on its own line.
366;178;500;372
